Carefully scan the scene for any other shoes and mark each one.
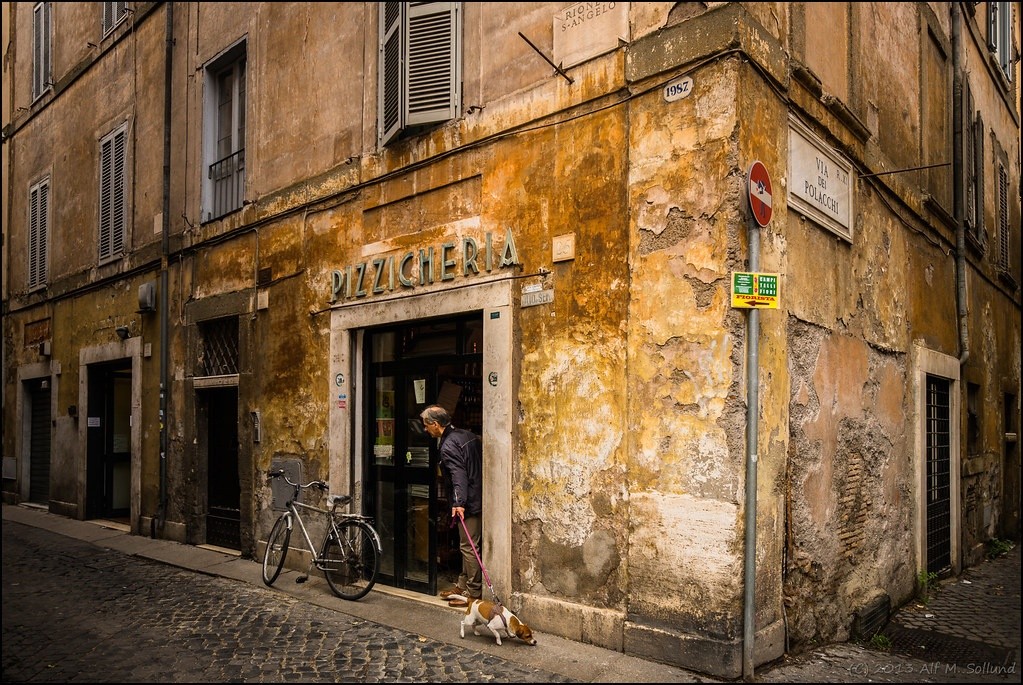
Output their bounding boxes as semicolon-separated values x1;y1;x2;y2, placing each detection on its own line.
449;591;473;607
440;583;466;599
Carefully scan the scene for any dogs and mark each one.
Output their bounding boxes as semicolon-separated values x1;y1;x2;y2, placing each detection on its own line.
446;587;540;650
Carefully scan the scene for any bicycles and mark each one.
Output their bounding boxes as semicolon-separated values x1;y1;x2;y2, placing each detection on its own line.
262;469;383;601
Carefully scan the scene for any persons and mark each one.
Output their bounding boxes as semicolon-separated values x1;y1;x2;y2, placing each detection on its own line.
420;405;482;606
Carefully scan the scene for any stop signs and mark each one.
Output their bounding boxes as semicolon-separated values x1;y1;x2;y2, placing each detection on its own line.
748;161;772;228
